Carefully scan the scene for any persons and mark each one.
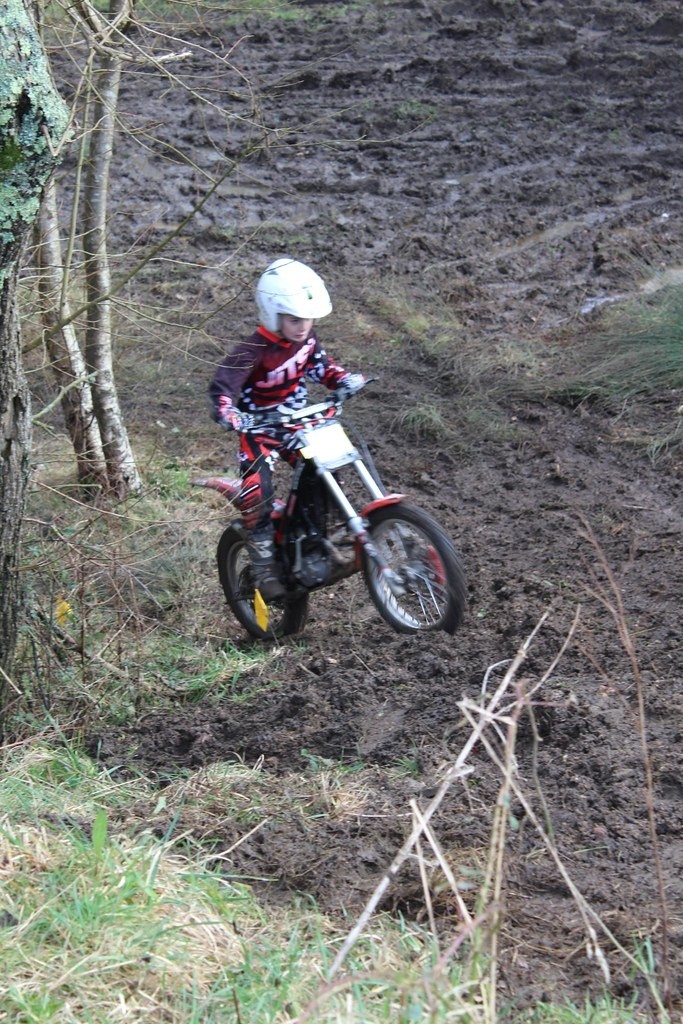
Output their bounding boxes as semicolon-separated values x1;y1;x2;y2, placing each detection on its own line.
210;259;365;601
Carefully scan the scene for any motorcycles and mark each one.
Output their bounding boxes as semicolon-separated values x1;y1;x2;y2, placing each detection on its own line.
188;374;467;641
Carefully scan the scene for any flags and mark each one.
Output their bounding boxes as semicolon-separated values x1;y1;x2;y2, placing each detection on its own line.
254;588;270;632
54;598;74;625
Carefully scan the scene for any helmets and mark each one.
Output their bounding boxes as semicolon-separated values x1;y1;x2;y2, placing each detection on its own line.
255;258;332;333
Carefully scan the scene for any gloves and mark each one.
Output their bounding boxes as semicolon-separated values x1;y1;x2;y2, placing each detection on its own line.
334;371;366;397
219;405;254;433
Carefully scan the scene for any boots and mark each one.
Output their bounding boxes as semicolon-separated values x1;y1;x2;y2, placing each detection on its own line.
244;523;286;604
